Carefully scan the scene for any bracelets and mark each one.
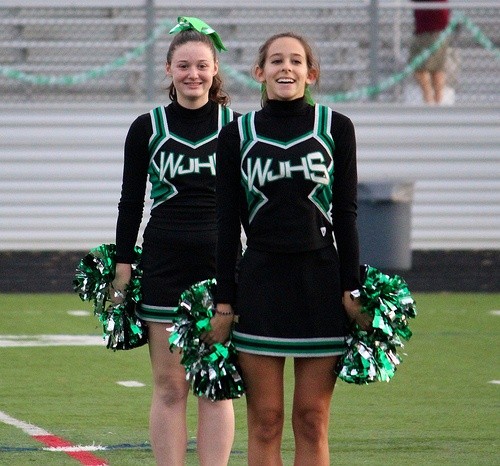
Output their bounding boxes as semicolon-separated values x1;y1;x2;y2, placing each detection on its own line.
216;309;234;315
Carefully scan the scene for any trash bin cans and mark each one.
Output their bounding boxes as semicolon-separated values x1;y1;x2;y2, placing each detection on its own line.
357;176;416;272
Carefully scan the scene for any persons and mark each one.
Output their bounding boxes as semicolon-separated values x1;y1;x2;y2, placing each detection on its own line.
199;32;374;466
411;0;453;104
103;16;244;466
